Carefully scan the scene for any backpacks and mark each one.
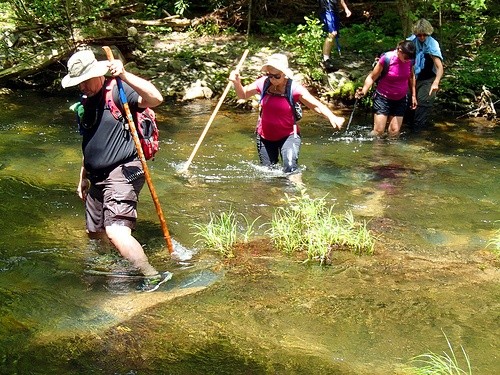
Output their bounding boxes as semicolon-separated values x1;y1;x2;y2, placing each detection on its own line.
81;77;159;161
260;78;302;121
371;54;415;85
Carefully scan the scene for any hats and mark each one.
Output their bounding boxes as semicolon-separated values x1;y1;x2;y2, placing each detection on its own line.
261;53;294;80
61;49;110;89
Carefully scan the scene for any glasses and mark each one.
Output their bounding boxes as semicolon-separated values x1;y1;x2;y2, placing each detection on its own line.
267;73;281;79
417;34;427;37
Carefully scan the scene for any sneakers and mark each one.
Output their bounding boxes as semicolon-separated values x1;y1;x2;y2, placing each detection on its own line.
134;272;172;293
322;59;339;73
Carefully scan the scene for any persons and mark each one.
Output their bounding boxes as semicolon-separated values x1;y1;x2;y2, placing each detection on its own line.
229;53;345;186
395;18;444;134
321;0;352;73
355;39;418;140
60;45;170;293
